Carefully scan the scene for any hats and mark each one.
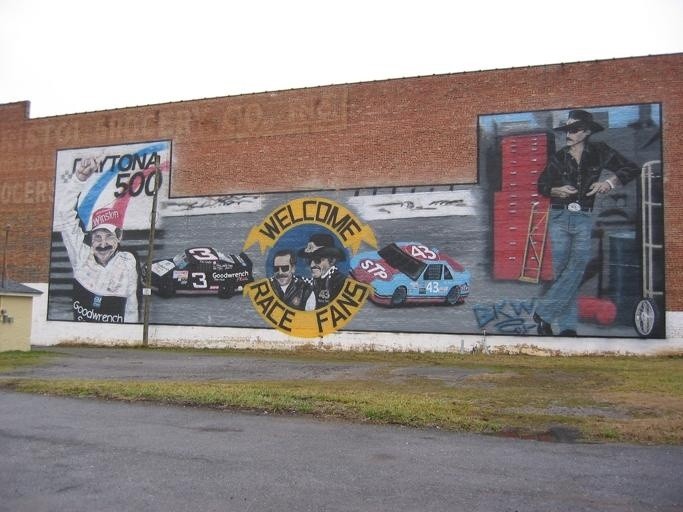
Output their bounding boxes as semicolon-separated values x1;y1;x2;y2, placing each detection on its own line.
298;234;346;262
551;110;604;131
92;208;122;233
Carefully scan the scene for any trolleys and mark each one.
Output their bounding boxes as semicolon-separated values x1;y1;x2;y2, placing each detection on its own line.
630;154;670;340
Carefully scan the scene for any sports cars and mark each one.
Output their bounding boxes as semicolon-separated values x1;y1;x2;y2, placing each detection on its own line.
140;246;255;299
346;240;470;308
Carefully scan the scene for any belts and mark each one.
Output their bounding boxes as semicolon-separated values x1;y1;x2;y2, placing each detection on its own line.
552;202;593;213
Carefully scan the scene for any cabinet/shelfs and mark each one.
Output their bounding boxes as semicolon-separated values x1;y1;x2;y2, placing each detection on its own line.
492;132;556;283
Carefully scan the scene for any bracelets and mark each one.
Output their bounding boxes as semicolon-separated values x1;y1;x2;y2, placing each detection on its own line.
605;179;615;192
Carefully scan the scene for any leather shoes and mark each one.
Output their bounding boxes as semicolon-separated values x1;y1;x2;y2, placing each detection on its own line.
560;330;578;337
533;313;552;335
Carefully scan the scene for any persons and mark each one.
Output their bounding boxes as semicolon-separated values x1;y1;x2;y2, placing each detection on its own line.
269;249;311;310
57;154;141;324
298;232;347;311
531;108;642;335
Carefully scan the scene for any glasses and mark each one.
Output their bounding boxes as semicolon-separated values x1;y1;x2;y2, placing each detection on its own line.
272;265;290;272
568;127;586;133
308;258;321;264
